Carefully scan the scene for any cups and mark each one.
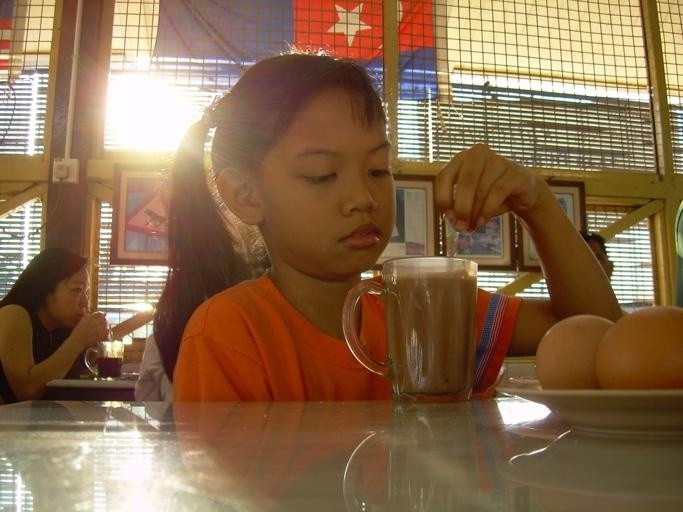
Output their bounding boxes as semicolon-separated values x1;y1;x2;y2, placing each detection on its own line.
83;339;125;381
341;255;478;404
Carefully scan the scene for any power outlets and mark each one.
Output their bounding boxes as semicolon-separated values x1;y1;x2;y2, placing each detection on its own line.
51;157;79;184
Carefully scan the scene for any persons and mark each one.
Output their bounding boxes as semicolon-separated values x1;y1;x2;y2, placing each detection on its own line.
152;45;623;404
132;334;174;401
3;248;111;401
579;231;613;283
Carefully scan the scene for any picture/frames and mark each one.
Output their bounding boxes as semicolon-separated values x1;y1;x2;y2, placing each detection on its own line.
373;174;439;271
517;180;585;271
110;163;174;266
442;211;516;273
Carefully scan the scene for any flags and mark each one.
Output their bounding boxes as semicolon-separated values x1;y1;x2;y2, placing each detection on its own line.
152;0;440;101
0;0;14;82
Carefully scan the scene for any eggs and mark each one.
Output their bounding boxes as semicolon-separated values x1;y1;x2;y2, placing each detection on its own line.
535;314;614;391
596;306;683;389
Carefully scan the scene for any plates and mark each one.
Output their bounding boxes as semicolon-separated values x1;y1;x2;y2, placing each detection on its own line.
120;372;140;380
494;380;683;437
